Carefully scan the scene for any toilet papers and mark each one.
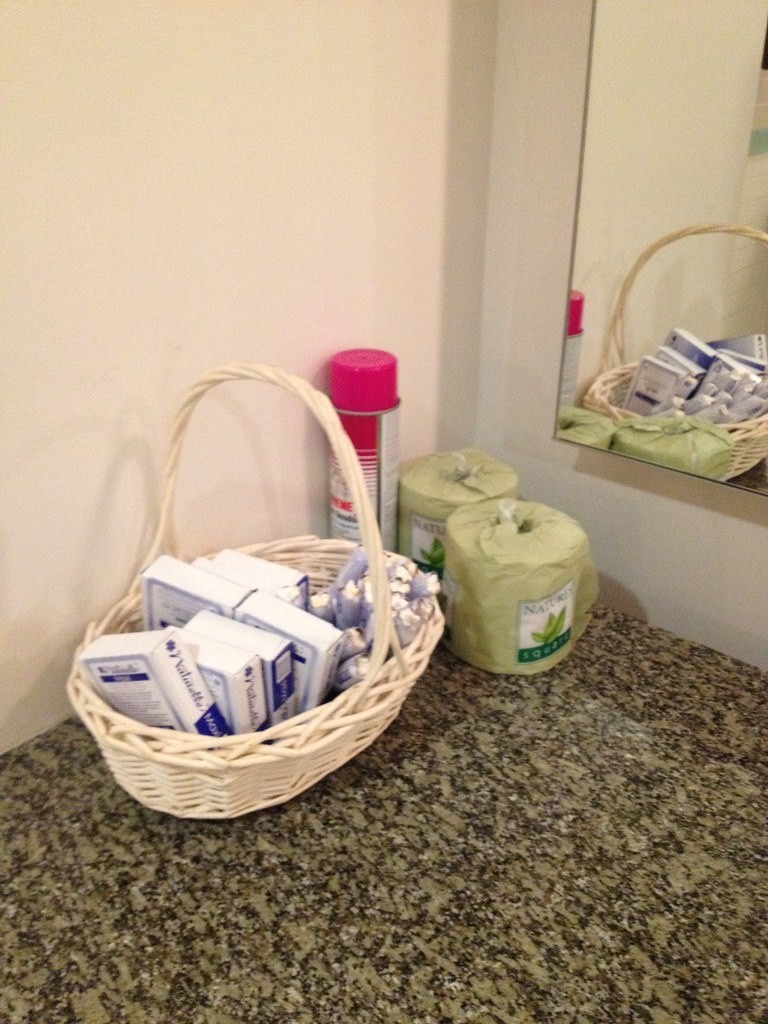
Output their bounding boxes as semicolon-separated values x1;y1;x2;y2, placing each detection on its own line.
615;407;733;484
554;403;619;453
396;450;529;596
441;495;603;681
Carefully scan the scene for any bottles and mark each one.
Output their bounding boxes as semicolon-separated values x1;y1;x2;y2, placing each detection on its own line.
326;347;404;555
554;287;587;409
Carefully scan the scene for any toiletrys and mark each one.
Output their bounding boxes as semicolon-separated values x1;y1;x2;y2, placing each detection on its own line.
619;311;768;451
73;532;449;764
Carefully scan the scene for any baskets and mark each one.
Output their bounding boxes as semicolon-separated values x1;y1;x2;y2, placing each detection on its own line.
583;219;768;481
66;364;448;819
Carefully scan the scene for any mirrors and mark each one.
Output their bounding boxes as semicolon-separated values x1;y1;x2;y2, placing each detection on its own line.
545;4;768;503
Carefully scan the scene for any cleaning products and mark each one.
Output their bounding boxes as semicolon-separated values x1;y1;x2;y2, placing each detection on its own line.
327;346;404;565
559;286;588;415
